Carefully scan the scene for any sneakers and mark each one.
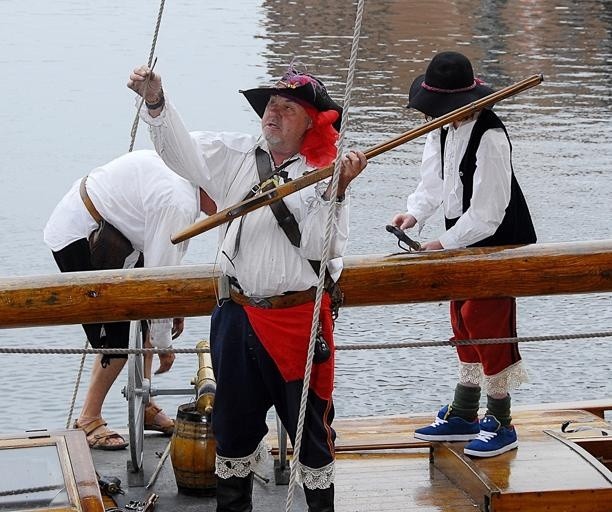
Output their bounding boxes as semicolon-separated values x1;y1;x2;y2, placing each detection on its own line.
464;415;519;457
413;403;480;441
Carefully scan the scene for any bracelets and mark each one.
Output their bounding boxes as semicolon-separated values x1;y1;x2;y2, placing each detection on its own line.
145;96;165;109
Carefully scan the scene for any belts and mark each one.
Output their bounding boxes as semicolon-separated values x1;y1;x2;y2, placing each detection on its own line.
78;175;100;226
230;287;316;310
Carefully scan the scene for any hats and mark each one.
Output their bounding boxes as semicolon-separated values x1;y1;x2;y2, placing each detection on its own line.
239;59;342;135
406;51;496;116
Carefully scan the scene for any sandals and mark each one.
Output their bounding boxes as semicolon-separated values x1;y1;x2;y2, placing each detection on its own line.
145;406;174;433
73;417;129;450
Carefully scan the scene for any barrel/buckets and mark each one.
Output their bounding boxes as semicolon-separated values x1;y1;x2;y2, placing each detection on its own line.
170;402;219;499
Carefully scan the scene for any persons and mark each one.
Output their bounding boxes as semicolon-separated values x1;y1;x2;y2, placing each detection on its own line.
41;148;217;450
125;65;367;512
391;52;537;457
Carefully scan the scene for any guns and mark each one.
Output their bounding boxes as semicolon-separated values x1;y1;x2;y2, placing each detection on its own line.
169;73;545;245
